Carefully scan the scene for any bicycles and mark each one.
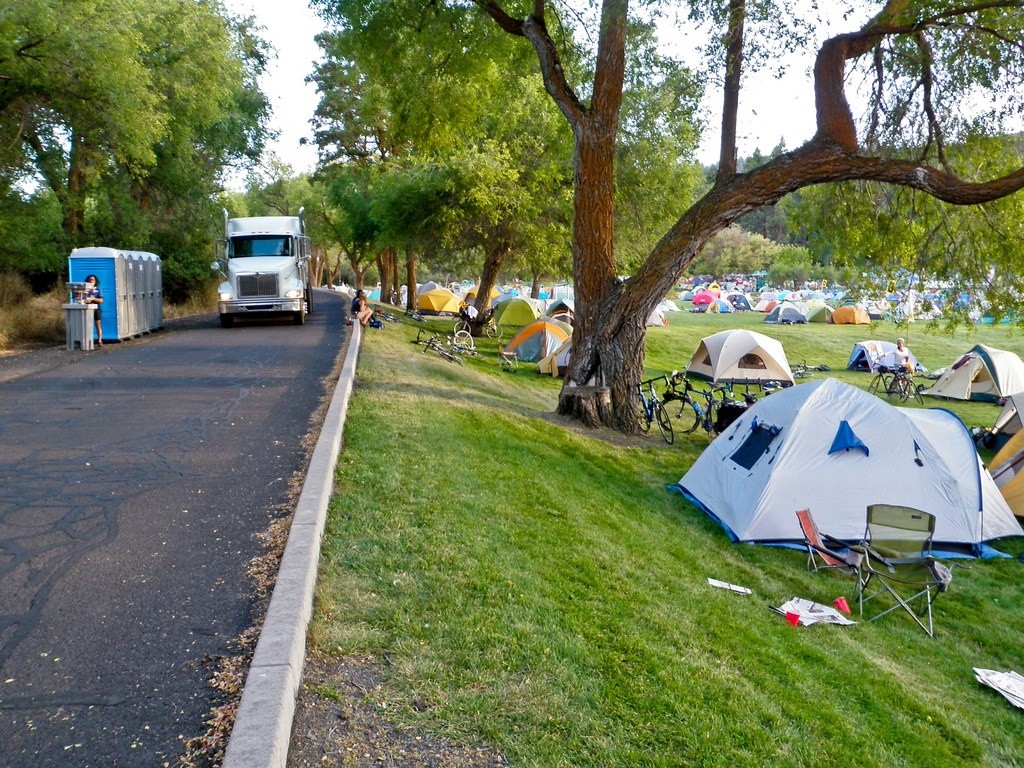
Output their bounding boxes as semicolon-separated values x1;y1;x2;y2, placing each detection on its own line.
867;361;924;406
635;374;673;446
740;357;829;406
372;302;403;324
657;363;739;439
405;307;429;323
414;305;504;367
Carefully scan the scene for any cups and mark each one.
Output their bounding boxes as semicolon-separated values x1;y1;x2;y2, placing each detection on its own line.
833;596;852;614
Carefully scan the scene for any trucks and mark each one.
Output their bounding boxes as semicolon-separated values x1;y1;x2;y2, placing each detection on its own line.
211;205;312;326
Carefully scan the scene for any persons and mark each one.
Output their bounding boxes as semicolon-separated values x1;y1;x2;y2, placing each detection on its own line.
353;289;363;303
875;338;909;370
83;274;104;346
351;291;373;327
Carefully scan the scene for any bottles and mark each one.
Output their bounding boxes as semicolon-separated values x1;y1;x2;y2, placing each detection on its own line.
647;398;652;412
702;403;710;412
693;401;702;415
714;399;721;411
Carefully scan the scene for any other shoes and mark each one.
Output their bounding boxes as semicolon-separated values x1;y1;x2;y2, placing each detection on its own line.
98;341;103;347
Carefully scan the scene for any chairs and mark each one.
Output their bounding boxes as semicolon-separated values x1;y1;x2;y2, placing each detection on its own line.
498;340;518;368
795;508;869;585
849;504;972;637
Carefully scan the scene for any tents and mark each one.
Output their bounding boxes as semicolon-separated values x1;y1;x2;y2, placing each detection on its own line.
684;329;796;388
667;375;1024;560
919;343;1024;406
417;281;459;313
460;285;538;326
846;341;928;372
504;314;574;379
646;299;681;327
868;280;1012;324
369;289;380;302
535;287;574;316
679;272;872;324
